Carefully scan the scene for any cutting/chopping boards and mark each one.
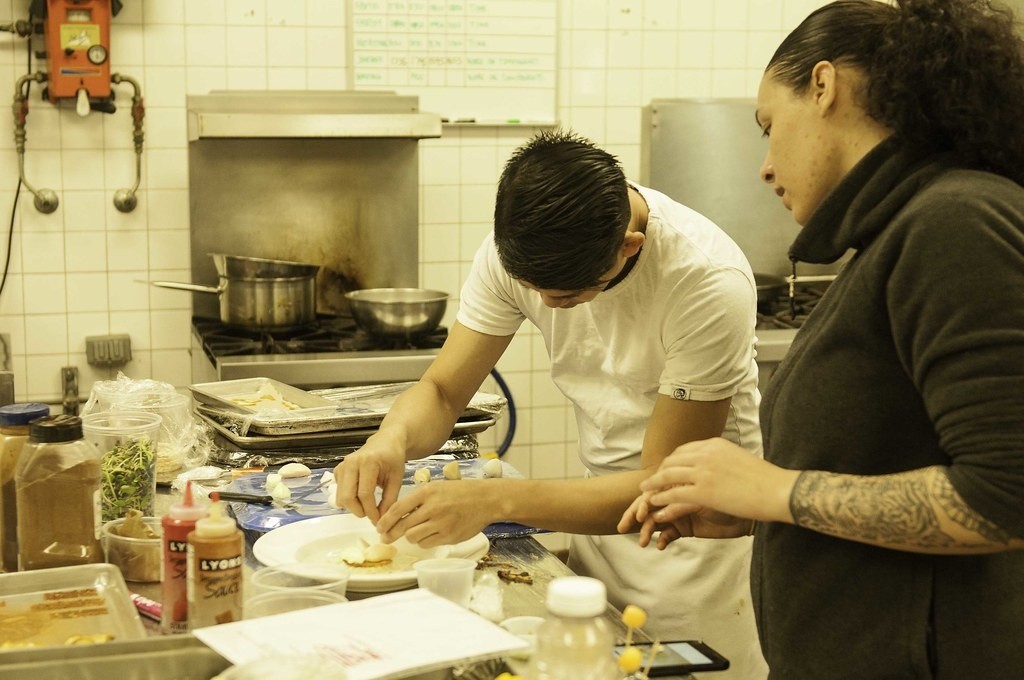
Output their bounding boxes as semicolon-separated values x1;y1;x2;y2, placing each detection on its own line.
227;458;570;538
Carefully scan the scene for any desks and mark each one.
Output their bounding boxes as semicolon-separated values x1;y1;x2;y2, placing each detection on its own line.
126;481;697;679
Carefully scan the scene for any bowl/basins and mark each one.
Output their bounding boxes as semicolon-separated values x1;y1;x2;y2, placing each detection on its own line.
102;514;165;582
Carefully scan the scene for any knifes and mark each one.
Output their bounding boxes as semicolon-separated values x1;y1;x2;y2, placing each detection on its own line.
209;489;298;512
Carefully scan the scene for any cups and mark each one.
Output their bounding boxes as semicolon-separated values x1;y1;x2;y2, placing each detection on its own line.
249;561;350;600
242;589;350;619
81;411;163;540
497;616;549;679
414;559;477;611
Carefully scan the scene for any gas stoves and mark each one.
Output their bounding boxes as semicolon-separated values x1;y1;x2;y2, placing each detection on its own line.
186;312;481;384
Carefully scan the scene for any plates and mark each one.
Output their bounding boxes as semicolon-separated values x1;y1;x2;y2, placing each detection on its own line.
183;376;337;415
187;587;534;680
191;588;535;680
252;510;491;591
0;564;151;648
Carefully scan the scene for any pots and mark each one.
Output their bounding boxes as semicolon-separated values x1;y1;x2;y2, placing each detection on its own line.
132;251;323;324
755;272;790;307
344;286;450;336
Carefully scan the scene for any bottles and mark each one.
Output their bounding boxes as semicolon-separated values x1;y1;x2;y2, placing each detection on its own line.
1;403;48;577
534;576;628;680
160;485;203;634
16;414;102;572
186;491;244;633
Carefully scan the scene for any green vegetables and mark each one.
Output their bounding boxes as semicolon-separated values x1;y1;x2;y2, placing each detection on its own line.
96;436;154;524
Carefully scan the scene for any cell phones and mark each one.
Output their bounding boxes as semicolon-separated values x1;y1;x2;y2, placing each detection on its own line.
613;640;730;677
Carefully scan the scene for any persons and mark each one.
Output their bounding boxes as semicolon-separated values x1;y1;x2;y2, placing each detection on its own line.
617;0;1024;680
333;138;770;680
